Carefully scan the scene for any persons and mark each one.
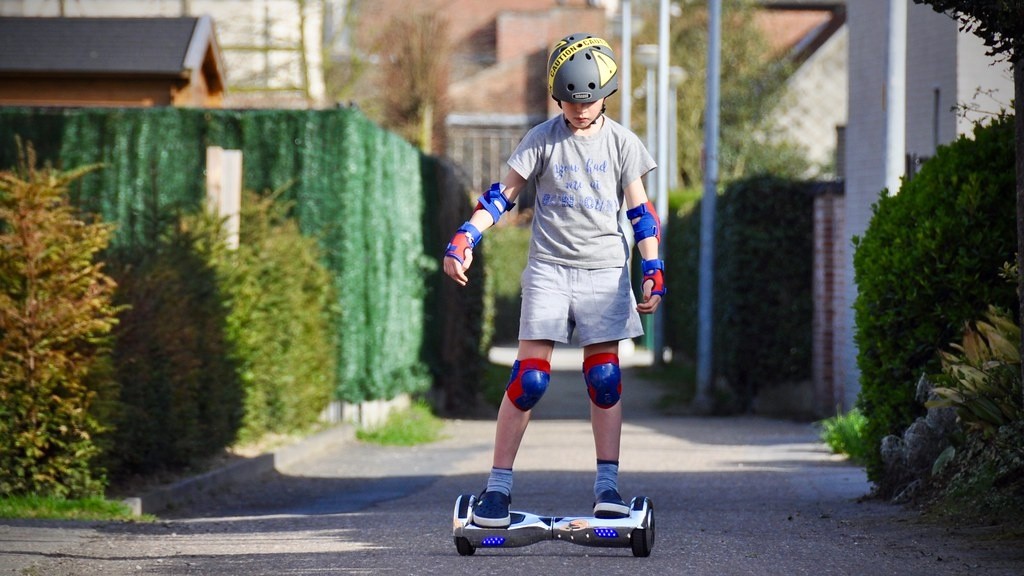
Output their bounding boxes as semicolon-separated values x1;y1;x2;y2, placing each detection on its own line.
444;32;668;527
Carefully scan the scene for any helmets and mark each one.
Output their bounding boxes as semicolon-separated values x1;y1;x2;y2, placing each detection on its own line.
546;33;622;103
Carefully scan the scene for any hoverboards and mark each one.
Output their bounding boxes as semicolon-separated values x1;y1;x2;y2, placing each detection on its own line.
453;494;655;558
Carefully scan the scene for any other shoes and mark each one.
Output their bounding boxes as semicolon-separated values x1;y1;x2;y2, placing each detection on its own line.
593;490;631;517
472;488;513;527
